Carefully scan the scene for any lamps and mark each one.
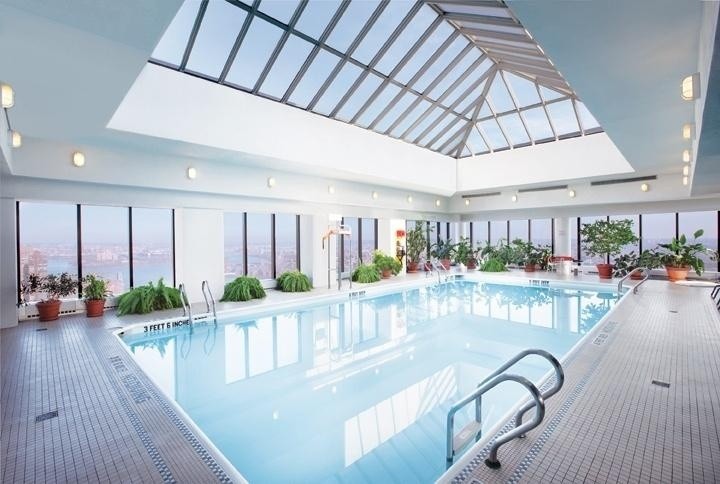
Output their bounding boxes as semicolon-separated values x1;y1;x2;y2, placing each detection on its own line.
12;132;22;149
408;196;413;203
375;194;377;198
680;166;692;185
269;178;275;185
73;152;87;167
188;168;196;177
682;124;692;139
2;85;15;109
641;184;649;191
464;200;470;204
681;75;699;102
568;191;576;199
329;186;335;193
437;201;441;205
512;195;519;201
683;150;693;163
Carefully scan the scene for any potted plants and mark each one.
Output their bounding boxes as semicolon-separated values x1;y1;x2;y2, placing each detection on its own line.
80;272;114;317
613;247;669;279
580;220;642;279
372;221;554;279
27;273;77;321
656;229;720;280
